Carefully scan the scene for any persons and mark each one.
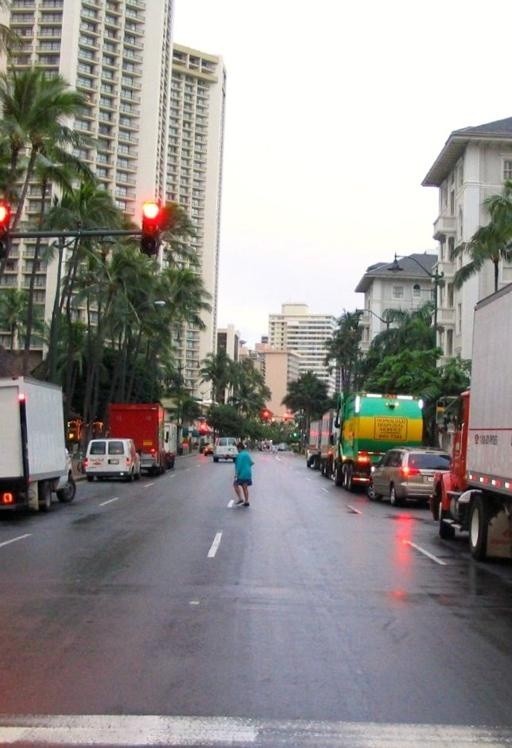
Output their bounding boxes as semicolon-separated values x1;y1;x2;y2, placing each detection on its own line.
230;440;255;508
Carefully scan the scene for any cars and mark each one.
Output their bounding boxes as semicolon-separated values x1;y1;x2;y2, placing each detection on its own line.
366;445;452;505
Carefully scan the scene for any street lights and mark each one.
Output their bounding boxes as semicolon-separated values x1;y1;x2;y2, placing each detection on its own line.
386;253;444;445
344;299;394;357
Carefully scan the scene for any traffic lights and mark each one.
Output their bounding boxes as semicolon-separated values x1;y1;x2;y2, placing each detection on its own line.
260;408;276;424
140;203;175;258
0;197;14;259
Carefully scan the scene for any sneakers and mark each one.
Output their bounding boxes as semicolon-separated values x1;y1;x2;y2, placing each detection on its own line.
236;500;249;506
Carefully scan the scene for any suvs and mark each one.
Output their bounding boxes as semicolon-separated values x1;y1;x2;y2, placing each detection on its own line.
83;438;142;482
213;437;239;462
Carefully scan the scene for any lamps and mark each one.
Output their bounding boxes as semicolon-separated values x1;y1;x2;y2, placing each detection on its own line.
387;251;443;279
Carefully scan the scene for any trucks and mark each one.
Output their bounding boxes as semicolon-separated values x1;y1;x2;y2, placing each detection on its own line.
304;390;426;492
428;282;511;561
108;403;177;475
1;374;77;513
